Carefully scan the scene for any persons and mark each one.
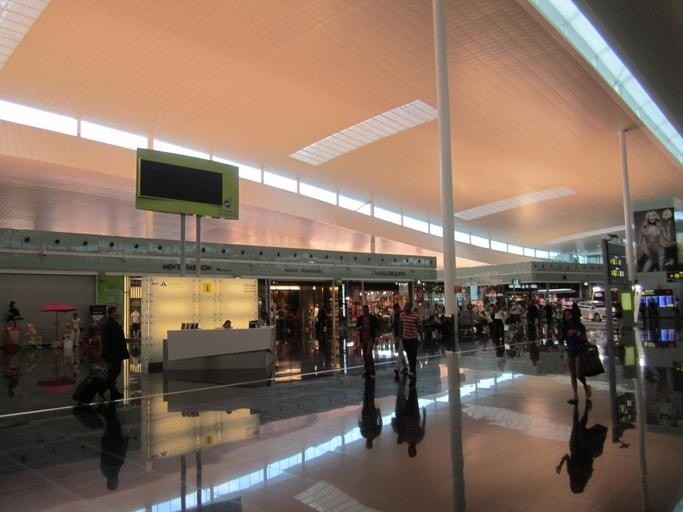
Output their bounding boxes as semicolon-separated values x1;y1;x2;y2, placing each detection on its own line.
555;308;592;404
555;399;600;494
357;378;383;450
390;374;408;444
610;296;682;337
638;210;673;272
221;319;232;329
403;374;426;457
99;305;134;401
130;308;142;338
259;295;582;379
71;311;81;346
96;401;130;491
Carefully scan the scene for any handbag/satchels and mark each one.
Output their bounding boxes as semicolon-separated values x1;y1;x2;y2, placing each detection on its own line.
580;344;605;376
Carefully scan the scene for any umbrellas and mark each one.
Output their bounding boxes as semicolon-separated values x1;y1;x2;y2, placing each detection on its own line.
37;303;76;347
36;355;76;393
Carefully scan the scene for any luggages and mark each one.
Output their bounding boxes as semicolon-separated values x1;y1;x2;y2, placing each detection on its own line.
71;361;113;405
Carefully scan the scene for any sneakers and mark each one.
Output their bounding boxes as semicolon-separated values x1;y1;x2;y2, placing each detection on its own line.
585;385;593;397
568;399;578;403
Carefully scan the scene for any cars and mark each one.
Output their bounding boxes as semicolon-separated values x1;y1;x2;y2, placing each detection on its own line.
589;331;619;348
575;300;616;322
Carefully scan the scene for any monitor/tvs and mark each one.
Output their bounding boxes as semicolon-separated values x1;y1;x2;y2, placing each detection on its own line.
248;320;260;328
181;323;198;329
